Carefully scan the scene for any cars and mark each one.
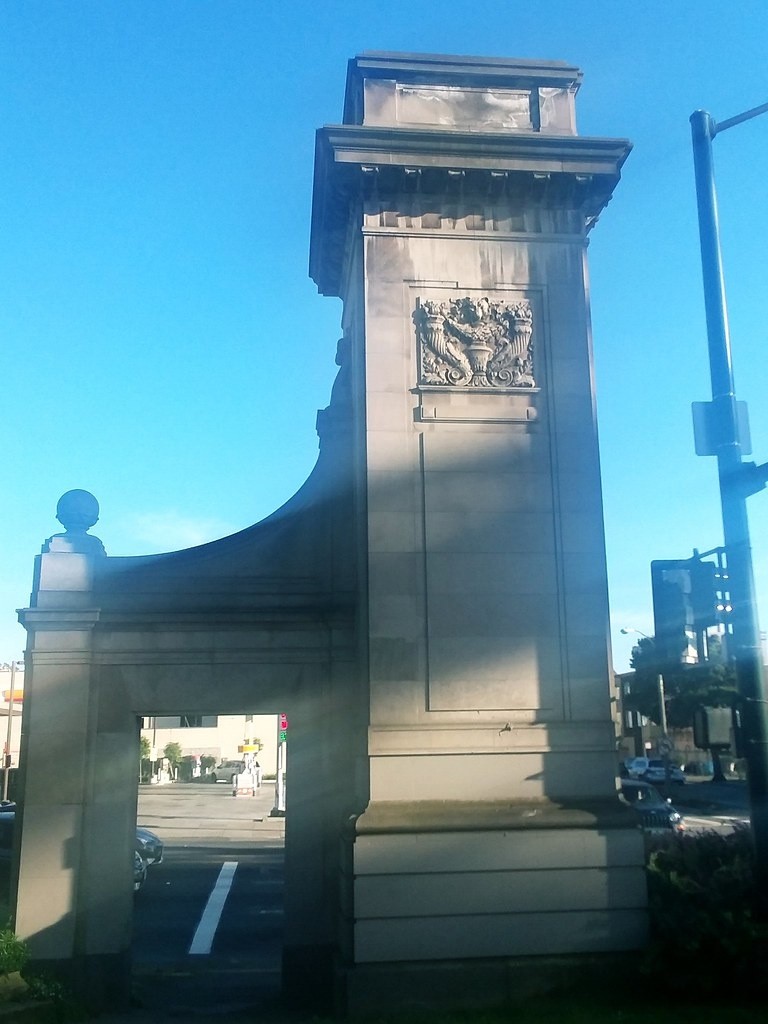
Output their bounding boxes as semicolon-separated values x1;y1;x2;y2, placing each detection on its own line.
623;754;687;783
136;827;166;868
209;759;263;784
0;810;147;897
617;777;683;835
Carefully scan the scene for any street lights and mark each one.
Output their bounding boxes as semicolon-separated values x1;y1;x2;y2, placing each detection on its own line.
618;625;667;738
